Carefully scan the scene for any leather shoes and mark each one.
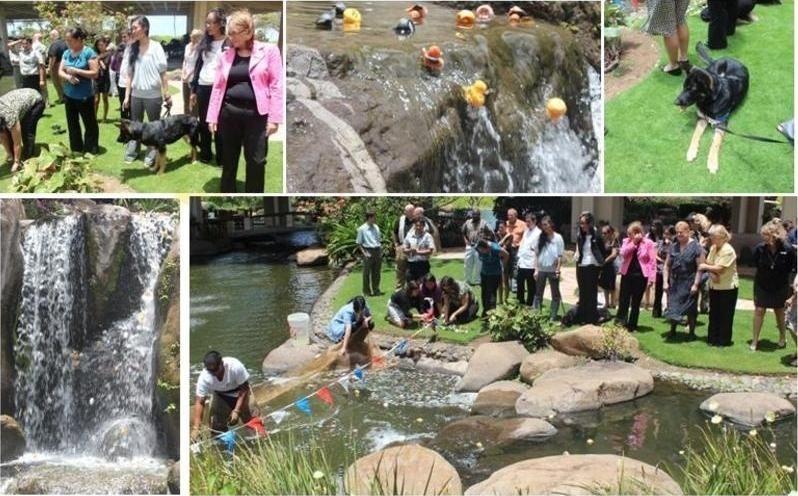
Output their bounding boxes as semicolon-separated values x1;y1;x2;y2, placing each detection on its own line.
658;60;691;75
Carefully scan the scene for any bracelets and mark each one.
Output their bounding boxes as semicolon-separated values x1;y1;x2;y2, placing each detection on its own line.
233;406;242;413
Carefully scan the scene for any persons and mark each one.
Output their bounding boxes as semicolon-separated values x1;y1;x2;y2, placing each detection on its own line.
192;350;266;448
322;294;377;361
1;8;228;175
208;10;283;191
355;210;386;298
384;198;797;369
647;0;783;77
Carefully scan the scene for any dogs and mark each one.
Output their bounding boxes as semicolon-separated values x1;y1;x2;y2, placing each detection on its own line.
672;40;750;175
113;113;201;176
555;306;616;328
